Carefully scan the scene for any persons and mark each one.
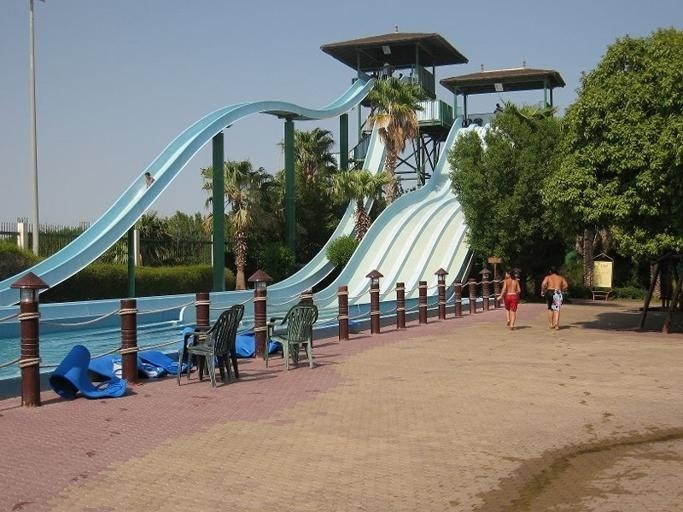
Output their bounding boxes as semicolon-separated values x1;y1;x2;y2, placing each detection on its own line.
540;267;568;330
493;101;503;113
497;270;520;329
144;172;154;189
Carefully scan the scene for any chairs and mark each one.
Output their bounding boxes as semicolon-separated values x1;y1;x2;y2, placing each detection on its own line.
175;310;237;388
196;302;245;381
265;303;318;371
